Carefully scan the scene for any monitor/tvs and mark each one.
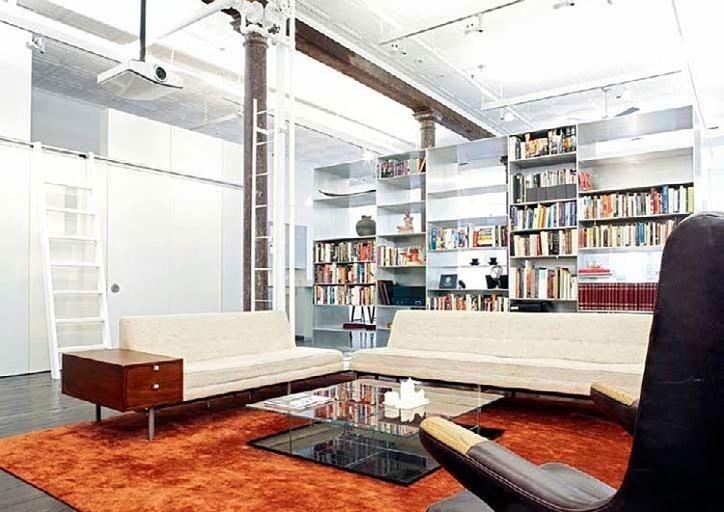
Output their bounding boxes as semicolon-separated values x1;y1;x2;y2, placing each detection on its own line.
510;299;554;312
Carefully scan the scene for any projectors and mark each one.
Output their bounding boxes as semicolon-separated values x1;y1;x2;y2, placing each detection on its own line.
96;59;184;101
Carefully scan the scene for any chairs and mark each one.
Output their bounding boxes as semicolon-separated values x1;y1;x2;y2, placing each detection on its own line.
415;210;724;511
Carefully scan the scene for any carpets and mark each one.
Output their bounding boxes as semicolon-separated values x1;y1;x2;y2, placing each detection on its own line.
1;375;636;512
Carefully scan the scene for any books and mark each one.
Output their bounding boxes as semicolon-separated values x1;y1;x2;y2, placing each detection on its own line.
510;201;577;230
378;158;426;178
314;240;376;305
510;265;576;299
578;185;694;247
429;224;508;249
510;168;577;202
578;282;657;311
509;126;577;160
377;246;403;266
510;229;578;256
426;292;508;312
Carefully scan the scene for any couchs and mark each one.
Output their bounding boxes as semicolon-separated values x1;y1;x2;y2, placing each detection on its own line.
339;302;654;409
113;309;351;422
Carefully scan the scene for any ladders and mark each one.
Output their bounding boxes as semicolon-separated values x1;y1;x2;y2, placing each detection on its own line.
33;142;114;381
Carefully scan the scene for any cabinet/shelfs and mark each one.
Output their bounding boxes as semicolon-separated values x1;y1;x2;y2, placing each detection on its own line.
57;346;185;414
304;104;700;354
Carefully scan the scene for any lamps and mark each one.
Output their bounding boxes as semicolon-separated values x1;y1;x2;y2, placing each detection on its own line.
388;12;485;56
